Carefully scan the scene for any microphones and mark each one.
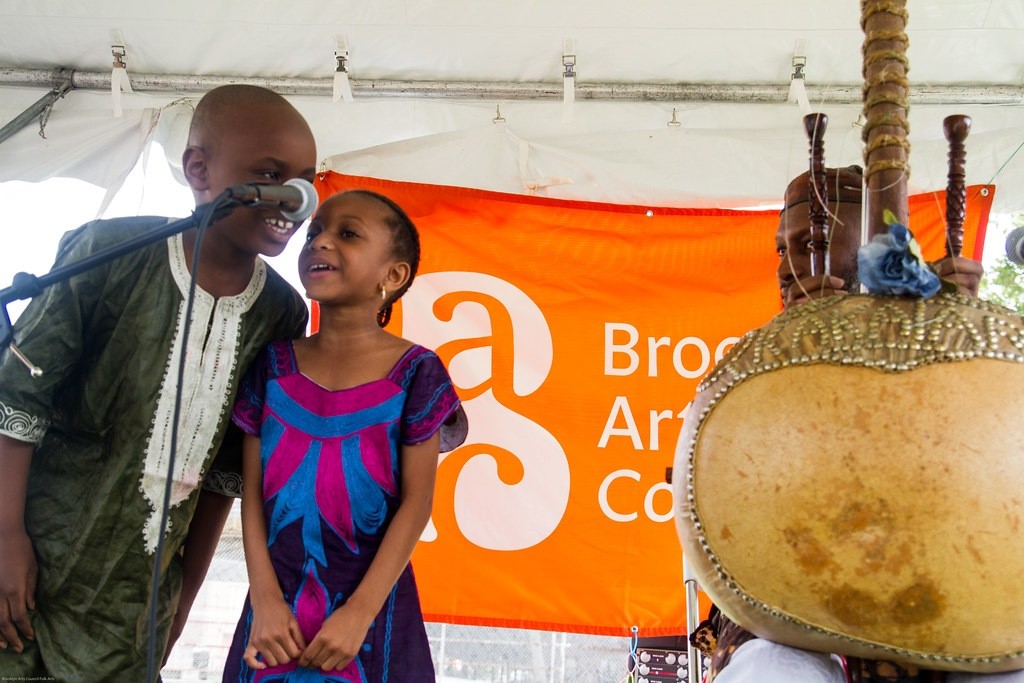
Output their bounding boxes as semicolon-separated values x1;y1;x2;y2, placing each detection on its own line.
226;178;318;221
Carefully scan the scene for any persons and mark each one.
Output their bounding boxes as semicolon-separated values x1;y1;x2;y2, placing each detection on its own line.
0;84;319;683
691;164;1024;683
220;190;467;683
